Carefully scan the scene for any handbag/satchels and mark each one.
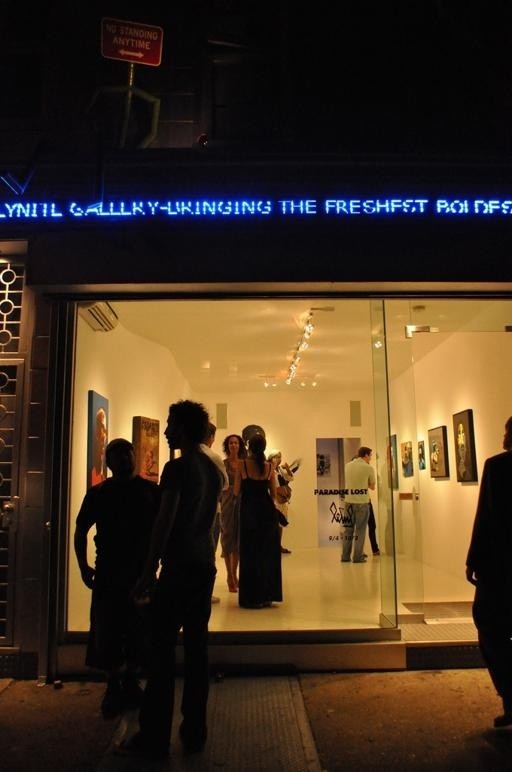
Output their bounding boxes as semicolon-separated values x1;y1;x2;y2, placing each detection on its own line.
275;485;291;505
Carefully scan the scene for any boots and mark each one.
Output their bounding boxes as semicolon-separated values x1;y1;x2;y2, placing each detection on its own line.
101;675;145;719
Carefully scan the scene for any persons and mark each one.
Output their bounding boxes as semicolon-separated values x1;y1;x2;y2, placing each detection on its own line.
75;435;162;720
352;456;380;557
112;396;223;763
463;417;510;731
388;421;472;487
339;446;376;563
200;420;293;610
91;406;107;485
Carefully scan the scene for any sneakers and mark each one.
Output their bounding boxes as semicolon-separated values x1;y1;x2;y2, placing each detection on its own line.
115;733;170;756
494;714;512;728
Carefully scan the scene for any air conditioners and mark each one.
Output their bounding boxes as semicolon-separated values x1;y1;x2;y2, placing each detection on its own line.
78;300;121;333
